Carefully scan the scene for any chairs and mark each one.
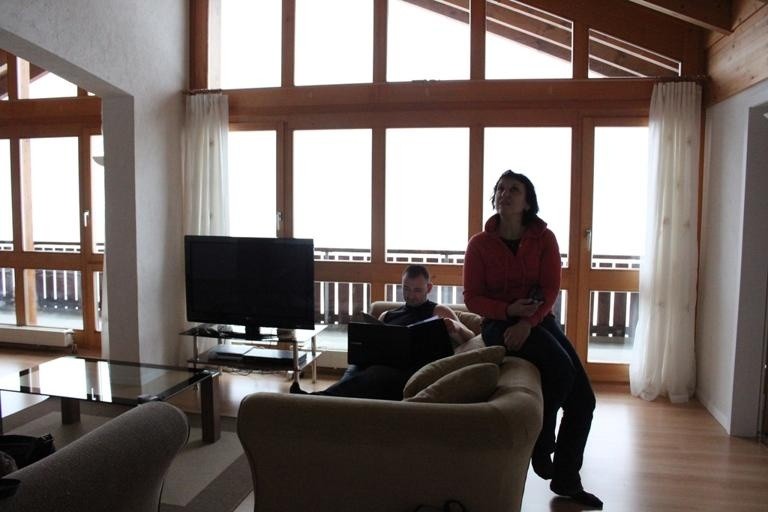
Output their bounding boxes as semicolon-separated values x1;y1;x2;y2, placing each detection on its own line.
1;399;193;512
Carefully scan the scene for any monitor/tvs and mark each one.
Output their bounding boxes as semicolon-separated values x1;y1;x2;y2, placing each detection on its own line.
184;235;314;338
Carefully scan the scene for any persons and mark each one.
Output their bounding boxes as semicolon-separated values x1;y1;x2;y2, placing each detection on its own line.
462;169;604;509
289;264;476;399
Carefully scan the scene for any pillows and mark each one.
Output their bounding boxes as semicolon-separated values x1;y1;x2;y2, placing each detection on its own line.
0;448;19;478
1;430;59;470
398;307;511;407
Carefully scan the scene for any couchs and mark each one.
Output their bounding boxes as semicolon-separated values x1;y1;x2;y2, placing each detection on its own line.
232;297;547;511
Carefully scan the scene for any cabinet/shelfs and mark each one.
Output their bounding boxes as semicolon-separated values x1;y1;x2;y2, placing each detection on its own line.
176;321;331;394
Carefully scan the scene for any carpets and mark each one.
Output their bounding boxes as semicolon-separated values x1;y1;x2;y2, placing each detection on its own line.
0;386;260;512
